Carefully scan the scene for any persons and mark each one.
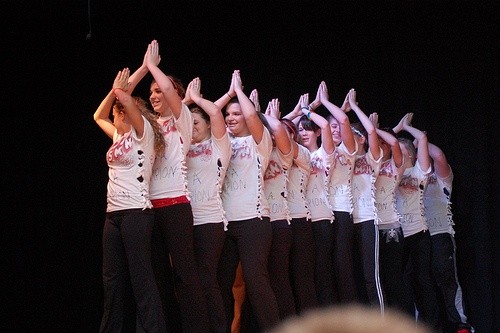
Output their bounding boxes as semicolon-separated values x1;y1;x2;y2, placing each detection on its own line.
367;110;408;321
244;79;388;321
119;38;211;333
412;130;472;333
180;76;234;333
91;66;165;333
212;69;281;333
389;111;442;332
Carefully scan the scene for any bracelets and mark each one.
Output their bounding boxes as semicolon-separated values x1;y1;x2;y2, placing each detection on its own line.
226;92;233;99
300;106;310;111
112;87;127;100
